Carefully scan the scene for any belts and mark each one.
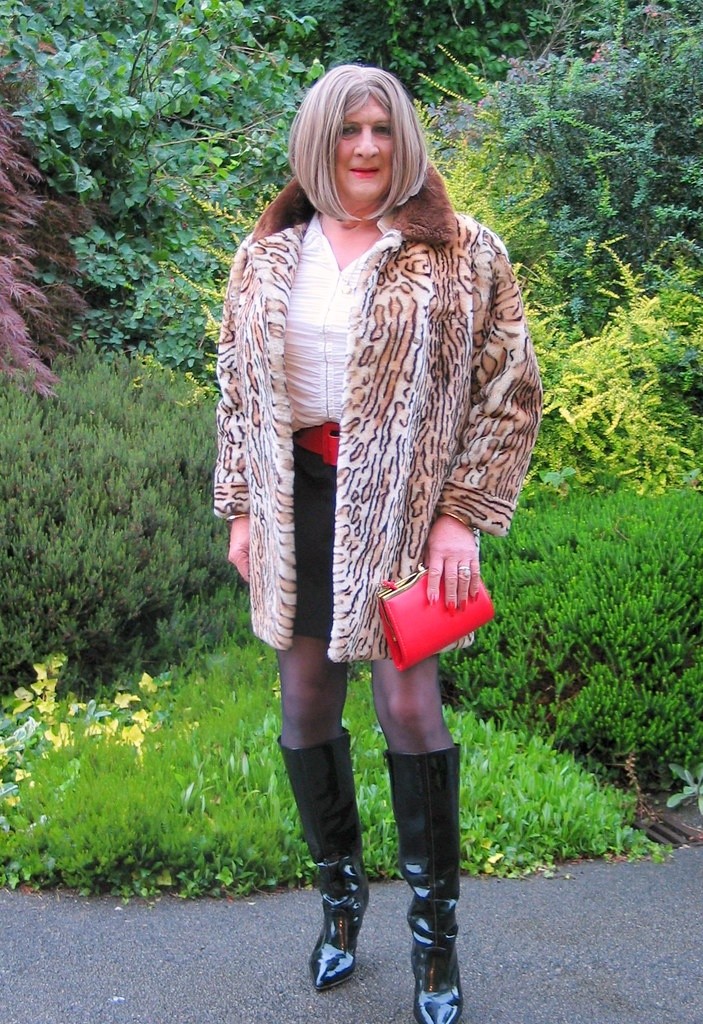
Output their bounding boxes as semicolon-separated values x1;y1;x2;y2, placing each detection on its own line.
296;422;341;465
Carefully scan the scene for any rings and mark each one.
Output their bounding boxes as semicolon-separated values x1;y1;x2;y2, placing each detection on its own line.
459;566;470;570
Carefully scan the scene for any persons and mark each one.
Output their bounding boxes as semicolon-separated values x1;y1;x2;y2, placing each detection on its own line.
215;66;542;1024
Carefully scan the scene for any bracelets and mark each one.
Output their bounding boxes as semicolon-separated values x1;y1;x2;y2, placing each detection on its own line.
441;510;474;534
225;514;251;522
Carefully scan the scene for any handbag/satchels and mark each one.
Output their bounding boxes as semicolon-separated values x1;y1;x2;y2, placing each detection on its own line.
377;562;495;671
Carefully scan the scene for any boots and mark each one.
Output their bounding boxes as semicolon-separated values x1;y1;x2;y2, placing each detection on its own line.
276;730;369;987
383;739;462;1024
